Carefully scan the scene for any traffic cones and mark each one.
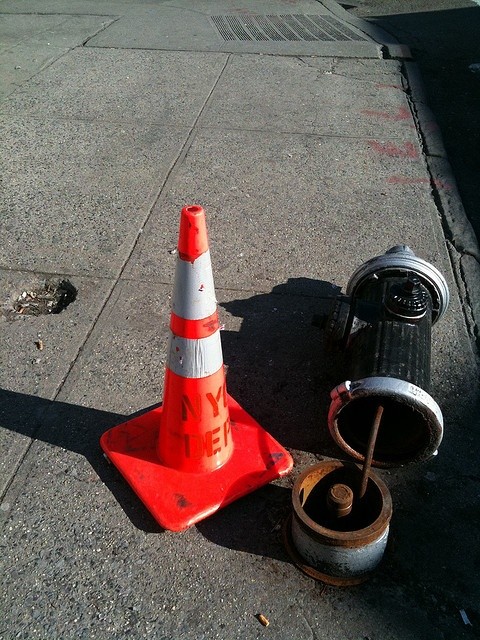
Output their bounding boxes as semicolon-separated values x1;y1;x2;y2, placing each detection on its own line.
100;205;293;532
